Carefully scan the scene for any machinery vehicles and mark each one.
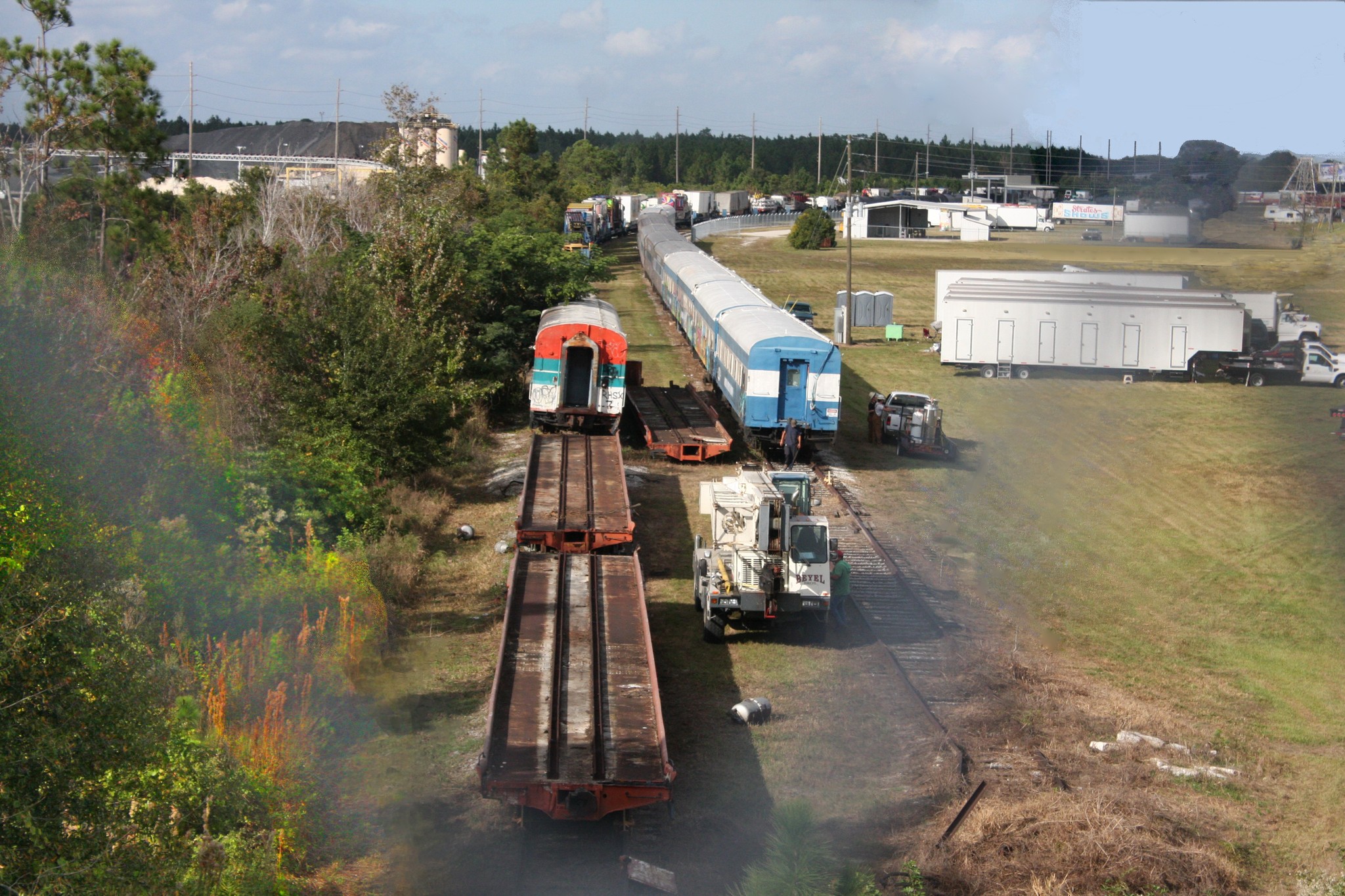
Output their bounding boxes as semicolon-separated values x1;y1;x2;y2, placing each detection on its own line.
690;460;839;644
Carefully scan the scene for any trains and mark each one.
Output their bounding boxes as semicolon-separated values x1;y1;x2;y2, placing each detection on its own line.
1235;191;1345;210
561;192;653;261
635;191;842;454
527;296;628;435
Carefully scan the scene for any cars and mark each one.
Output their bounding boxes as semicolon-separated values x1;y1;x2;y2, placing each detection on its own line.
1081;228;1103;241
1253;340;1298;358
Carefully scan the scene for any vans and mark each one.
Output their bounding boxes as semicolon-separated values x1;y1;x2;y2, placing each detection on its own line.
1275;210;1320;225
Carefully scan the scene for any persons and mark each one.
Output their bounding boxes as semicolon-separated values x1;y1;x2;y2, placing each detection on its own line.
867;391;897;447
1271;211;1344;231
780;420;801;471
862;187;869;197
792;527;826;563
829;550;851;634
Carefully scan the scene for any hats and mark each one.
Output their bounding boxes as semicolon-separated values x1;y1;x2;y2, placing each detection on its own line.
791;419;797;427
834;549;843;556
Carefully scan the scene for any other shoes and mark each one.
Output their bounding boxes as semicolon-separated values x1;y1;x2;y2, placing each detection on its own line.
784;464;788;470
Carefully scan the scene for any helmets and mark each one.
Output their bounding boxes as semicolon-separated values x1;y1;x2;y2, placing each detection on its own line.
869;392;875;400
877;394;885;399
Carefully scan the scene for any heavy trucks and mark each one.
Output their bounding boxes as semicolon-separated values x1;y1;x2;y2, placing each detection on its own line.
932;279;1321;340
987;206;1055;232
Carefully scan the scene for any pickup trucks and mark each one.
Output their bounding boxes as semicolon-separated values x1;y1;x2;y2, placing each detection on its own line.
779;301;818;326
881;391;931;435
1219;342;1345;389
